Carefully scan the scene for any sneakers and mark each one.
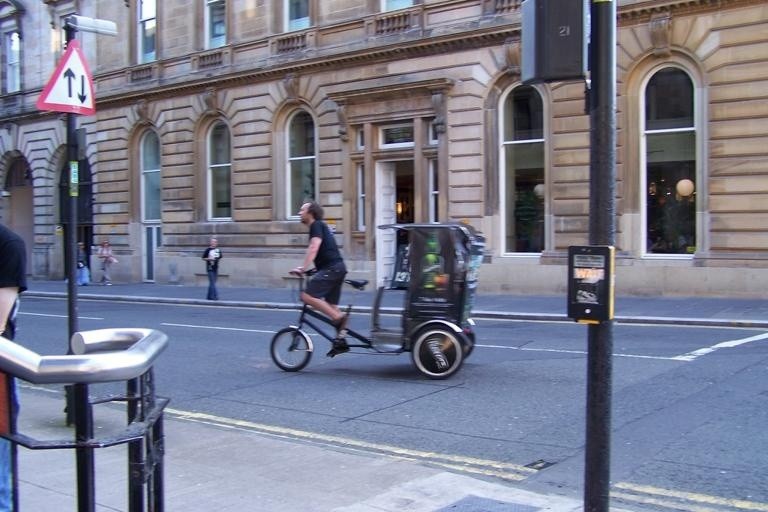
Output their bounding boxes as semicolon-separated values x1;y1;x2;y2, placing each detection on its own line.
333;315;348;336
328;342;348;357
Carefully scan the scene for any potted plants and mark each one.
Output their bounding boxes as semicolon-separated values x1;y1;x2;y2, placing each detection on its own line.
515;194;536;251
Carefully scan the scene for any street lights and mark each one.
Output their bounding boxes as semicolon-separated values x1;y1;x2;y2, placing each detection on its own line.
534;184;545;250
677;180;694;226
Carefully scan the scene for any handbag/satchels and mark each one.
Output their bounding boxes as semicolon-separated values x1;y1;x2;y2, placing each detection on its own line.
96;255;106;262
77;260;84;268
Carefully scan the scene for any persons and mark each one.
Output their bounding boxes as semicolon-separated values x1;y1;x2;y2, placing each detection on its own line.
201;239;223;300
290;202;350;356
0;223;28;511
97;239;113;285
77;242;90;286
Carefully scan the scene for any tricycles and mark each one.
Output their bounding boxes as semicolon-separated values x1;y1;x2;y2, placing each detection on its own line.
269;216;485;380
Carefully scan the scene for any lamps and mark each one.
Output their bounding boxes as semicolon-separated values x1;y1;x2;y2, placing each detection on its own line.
677;179;694;198
533;184;545;199
